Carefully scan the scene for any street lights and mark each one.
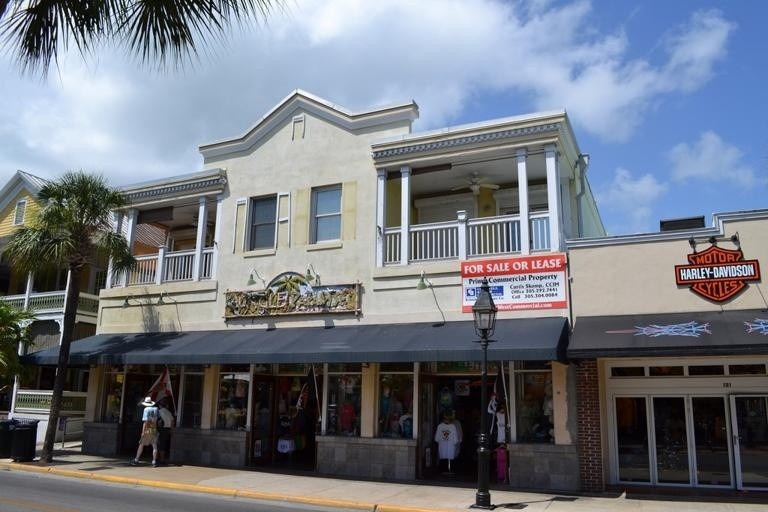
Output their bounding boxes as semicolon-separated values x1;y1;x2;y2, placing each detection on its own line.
467;273;501;512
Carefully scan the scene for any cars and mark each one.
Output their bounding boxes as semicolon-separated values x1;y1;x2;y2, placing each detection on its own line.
617;442;680;471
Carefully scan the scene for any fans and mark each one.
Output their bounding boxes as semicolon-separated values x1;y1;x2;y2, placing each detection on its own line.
450;170;501;197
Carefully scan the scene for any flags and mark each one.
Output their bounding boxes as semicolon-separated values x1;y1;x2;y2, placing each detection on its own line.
487;360;505;438
137;368;172;407
294;368;318;417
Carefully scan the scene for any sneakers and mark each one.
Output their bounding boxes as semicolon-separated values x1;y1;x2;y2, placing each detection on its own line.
151;460;159;467
129;460;138;466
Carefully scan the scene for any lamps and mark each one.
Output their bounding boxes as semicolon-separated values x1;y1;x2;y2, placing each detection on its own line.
247;269;267;287
156;289;177;306
120;294;142;309
303;262;323;286
417;271;447;321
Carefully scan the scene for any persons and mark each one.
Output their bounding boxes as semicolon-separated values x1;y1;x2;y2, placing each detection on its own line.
129;396;161;467
108;387;121;421
158;399;175;466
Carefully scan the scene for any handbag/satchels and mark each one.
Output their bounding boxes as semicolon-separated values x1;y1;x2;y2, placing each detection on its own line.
156;409;164;432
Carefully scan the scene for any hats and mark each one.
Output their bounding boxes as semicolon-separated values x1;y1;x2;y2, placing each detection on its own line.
141;396;156;407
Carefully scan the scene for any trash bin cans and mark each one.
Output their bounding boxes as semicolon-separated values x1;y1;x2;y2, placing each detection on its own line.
0;417;40;462
